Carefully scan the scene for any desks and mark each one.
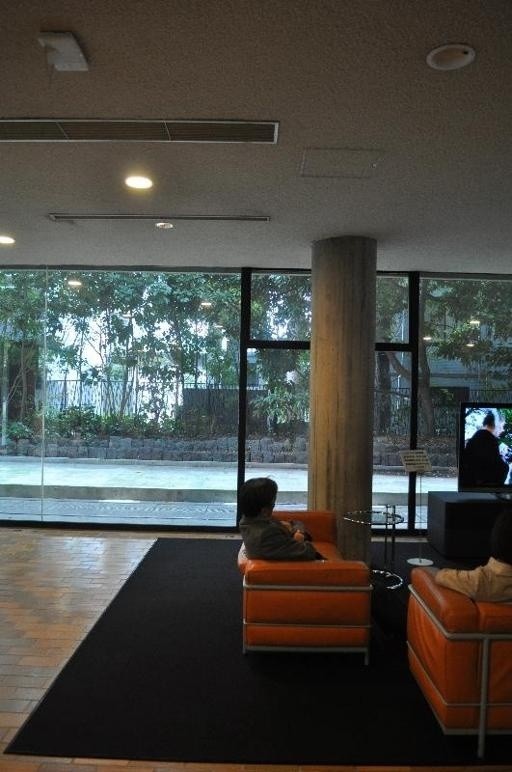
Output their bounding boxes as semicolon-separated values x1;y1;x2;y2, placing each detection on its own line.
428;491;510;556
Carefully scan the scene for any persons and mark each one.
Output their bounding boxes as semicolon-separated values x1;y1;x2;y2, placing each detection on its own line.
238;478;406;641
428;505;512;600
461;412;508;492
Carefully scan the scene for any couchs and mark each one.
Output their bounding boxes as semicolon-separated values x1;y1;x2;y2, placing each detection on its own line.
236;512;373;664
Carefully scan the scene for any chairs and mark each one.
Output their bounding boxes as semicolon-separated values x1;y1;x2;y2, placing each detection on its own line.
405;567;512;758
2;538;512;765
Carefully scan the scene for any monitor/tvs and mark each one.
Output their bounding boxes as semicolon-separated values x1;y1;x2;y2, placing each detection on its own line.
458;402;512;499
